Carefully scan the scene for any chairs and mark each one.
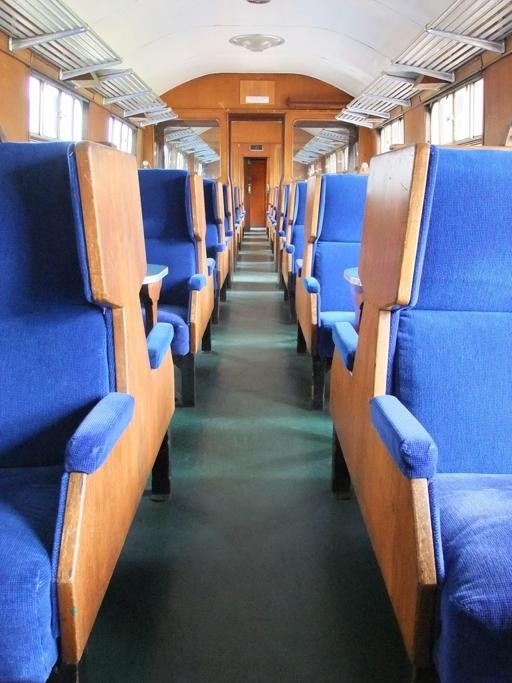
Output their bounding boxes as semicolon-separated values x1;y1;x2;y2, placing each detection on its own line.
0;140;245;682
265;144;510;683
